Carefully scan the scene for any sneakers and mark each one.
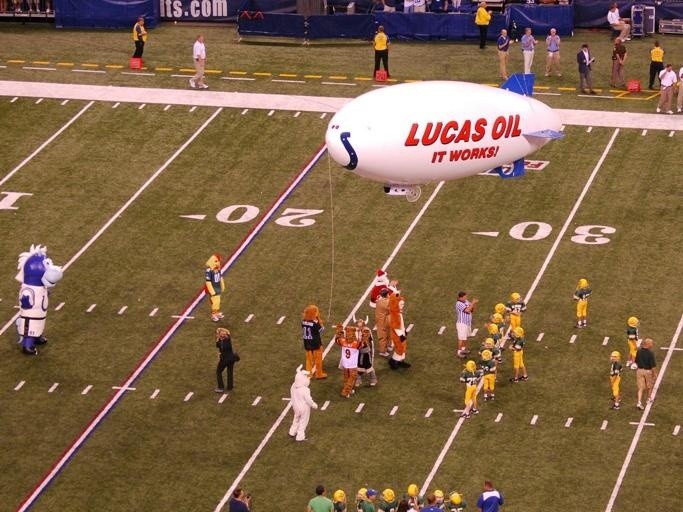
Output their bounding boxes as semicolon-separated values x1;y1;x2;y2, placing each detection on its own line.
215;387;224;394
574;320;588;330
624;360;637;371
482;393;496;403
460;408;480;420
210;311;225;322
543;72;625;95
189;77;209;92
656;106;683;115
610;395;624;410
635;400;646;411
510;374;529;385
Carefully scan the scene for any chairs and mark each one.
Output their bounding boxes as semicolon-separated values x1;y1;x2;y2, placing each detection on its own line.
611;25;620;39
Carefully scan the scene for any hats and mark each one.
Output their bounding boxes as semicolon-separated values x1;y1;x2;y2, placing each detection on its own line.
366;489;378;497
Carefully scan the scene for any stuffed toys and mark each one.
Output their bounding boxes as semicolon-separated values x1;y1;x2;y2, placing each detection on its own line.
202;253;226;322
300;303;328;379
12;243;64;356
333;269;415;399
287;363;319;442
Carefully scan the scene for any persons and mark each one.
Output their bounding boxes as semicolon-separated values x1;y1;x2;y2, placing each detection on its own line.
606;4;632;43
576;44;597;95
607;38;628;90
609;1;630;41
229;486;252;511
371;25;392;78
306;483;466;512
676;67;682;114
473;1;494;50
496;28;510;81
608;350;624;410
572;277;594;329
634;338;658;410
453;291;529;419
475;479;504;512
647;40;665;89
656;64;678;115
188;34;209;90
519;27;540;75
372;0;462;14
131;18;148;63
544;28;562;78
213;326;236;393
625;316;641;370
0;0;52;16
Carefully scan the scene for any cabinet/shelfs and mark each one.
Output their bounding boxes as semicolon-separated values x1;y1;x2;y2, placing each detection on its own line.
658;19;683;35
631;4;656;38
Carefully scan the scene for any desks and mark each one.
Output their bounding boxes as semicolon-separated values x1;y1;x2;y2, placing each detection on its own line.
504;3;574;36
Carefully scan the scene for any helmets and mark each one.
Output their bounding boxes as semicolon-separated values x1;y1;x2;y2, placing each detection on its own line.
610;350;622;364
433;489;445;502
466;360;476;372
513;326;525;338
626;315;641;331
407;483;420;496
448;491;463;504
493;313;504;323
484;338;495;348
509;291;523;304
481;349;493;362
381;489;396;503
487;323;500;336
577;276;589;290
357;487;367;499
494;303;508;314
333;488;346;503
